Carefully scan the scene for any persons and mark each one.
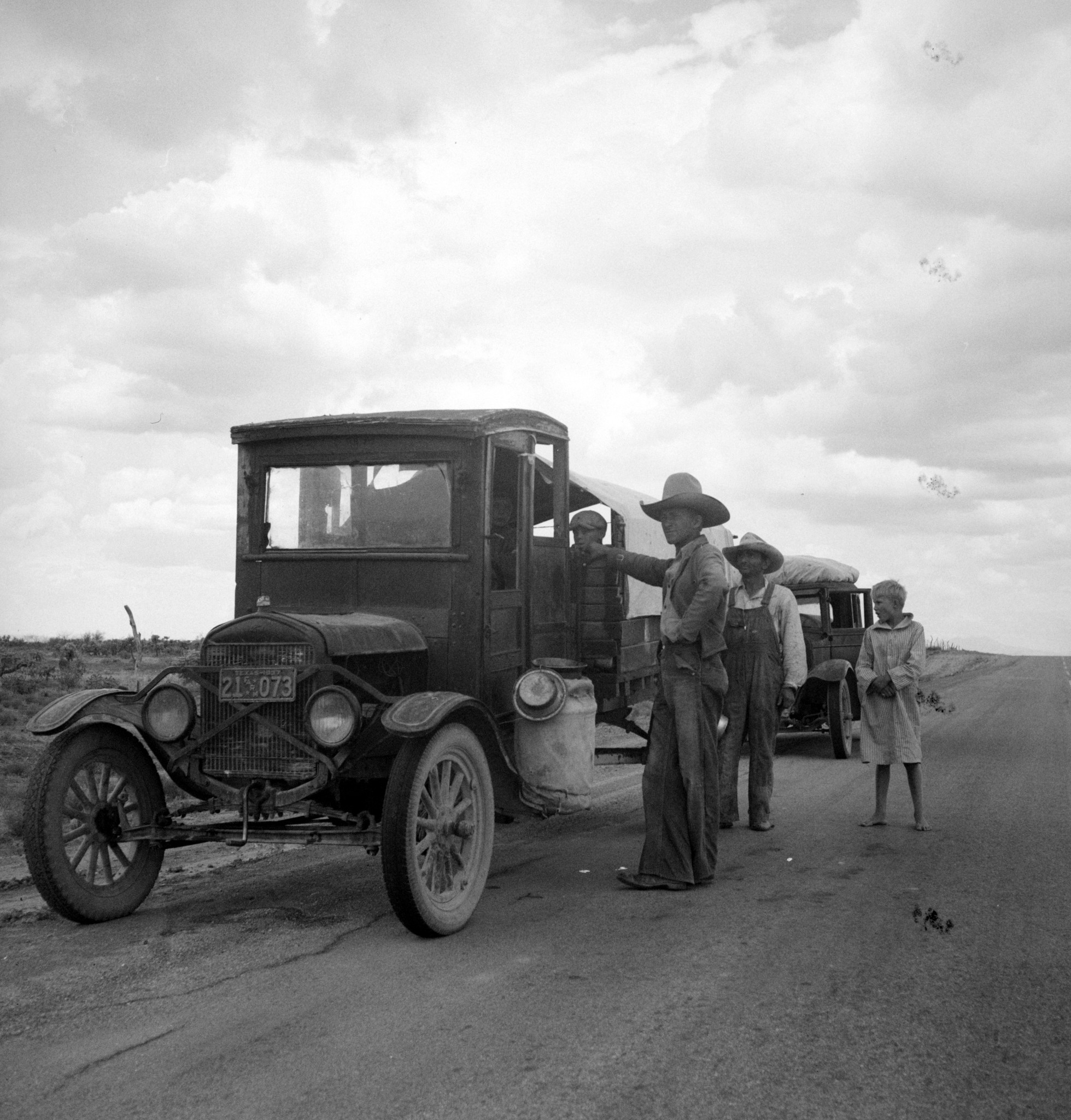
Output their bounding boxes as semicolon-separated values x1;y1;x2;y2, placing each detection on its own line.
573;472;729;889
716;532;807;831
569;510;607;545
855;581;930;831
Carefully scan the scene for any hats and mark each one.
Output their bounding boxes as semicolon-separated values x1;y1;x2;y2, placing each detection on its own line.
640;472;730;528
568;510;607;531
722;532;784;574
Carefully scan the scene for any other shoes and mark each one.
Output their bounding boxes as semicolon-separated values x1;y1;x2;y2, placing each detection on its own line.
749;819;770;831
719;822;733;828
617;871;686;890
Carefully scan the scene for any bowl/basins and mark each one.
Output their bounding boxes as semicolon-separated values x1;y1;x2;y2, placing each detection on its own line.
531;657;588;679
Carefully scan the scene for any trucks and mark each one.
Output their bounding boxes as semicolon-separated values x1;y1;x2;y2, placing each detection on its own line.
21;408;660;940
741;581;874;759
